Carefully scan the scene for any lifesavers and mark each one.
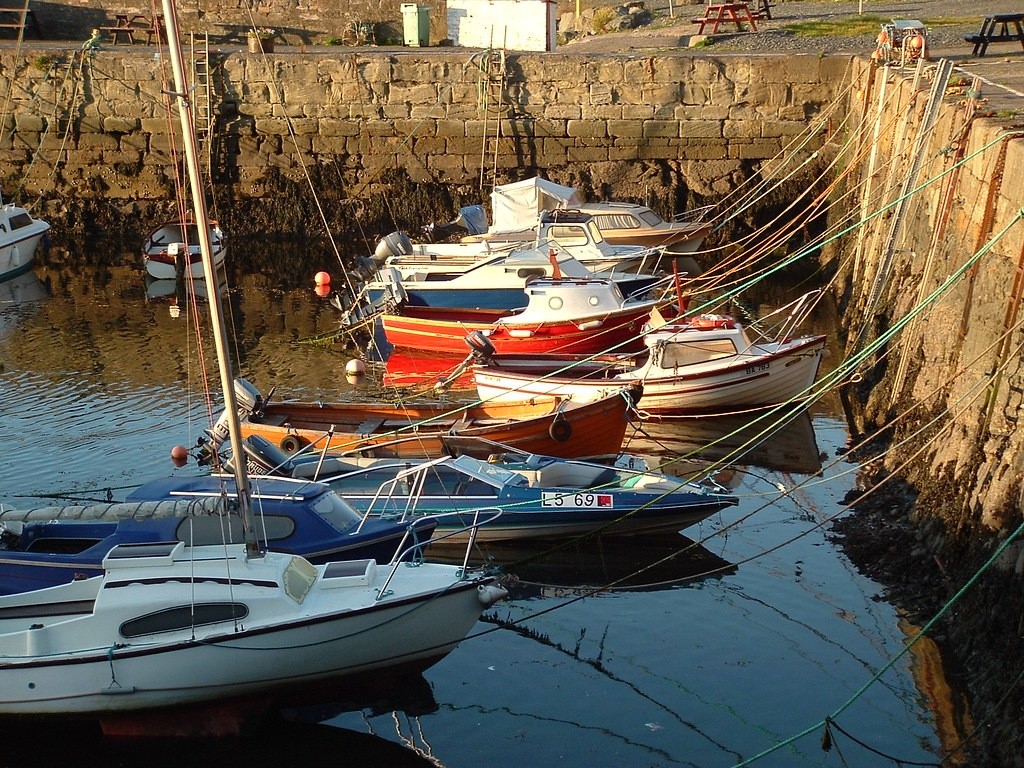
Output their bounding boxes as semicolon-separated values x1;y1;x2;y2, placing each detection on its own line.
692;313;736;330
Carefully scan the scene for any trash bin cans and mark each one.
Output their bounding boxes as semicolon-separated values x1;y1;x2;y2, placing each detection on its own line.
400;3;431;47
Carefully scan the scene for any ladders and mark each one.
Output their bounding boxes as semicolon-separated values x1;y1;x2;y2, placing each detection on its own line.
190;30;213;194
0;0;30;138
480;26;508;188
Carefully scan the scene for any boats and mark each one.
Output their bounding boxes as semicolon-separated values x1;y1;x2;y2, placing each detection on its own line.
1;200;50;283
141;209;228;280
1;0;832;735
